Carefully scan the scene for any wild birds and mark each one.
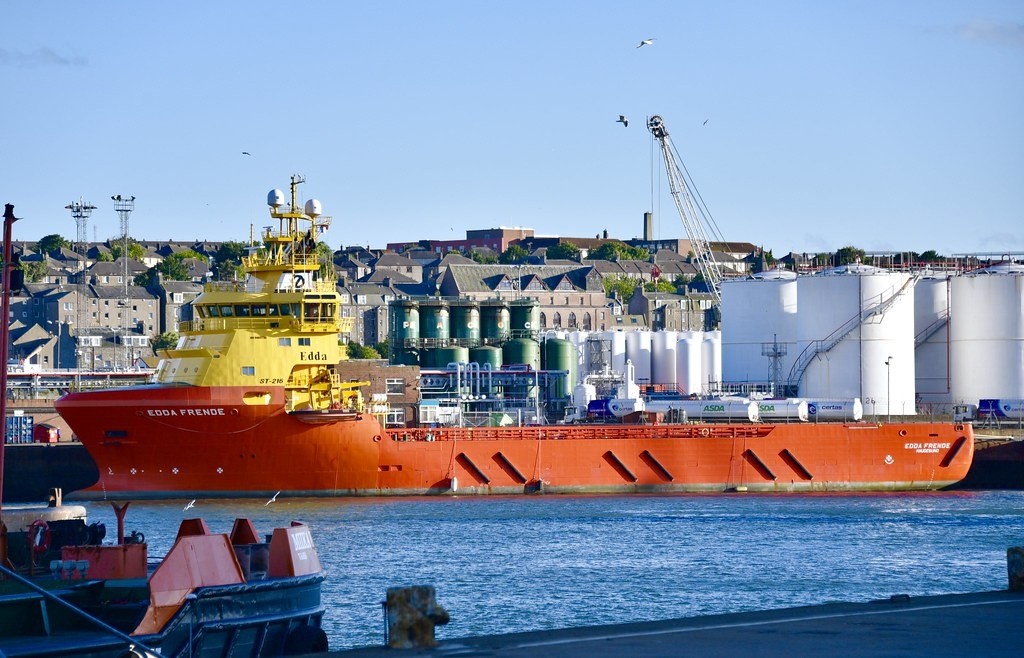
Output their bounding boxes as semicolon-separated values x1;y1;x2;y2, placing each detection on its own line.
263;490;281;507
182;500;196;512
616;115;629;127
637;38;654;48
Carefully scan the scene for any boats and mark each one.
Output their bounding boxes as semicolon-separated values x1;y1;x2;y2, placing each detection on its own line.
50;173;975;499
0;496;330;658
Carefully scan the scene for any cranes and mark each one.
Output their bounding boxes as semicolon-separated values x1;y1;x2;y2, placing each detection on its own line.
646;114;757;317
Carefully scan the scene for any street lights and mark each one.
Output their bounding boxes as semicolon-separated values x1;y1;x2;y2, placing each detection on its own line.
544;329;553;411
887;355;894;423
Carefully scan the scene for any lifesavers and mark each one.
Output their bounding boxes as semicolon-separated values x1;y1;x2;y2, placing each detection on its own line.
374;435;382;442
28;519;50;553
899;429;907;437
702;429;709;437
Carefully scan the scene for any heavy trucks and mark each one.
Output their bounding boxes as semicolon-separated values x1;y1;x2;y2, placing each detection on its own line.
562;398;865;424
952;398;1024;428
